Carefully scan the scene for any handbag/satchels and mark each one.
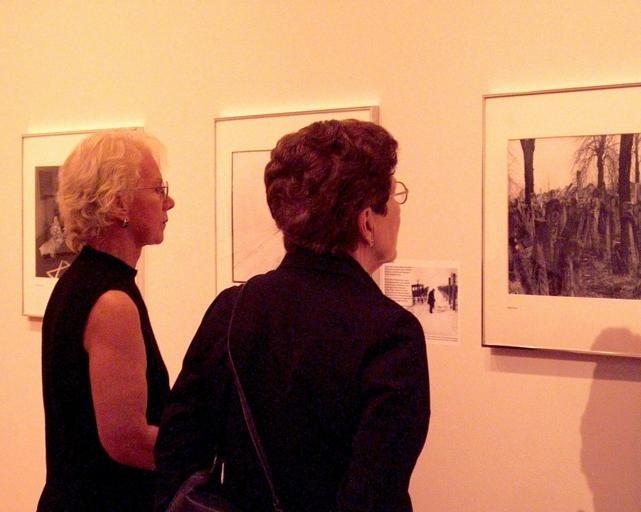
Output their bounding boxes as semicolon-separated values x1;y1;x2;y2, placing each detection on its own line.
167;469;287;512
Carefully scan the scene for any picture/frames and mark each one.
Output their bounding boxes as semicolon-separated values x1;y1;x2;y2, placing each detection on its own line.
481;83;641;362
213;105;380;299
21;125;147;321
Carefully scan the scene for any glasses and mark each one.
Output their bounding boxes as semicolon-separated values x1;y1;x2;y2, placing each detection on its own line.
391;182;407;204
120;181;169;200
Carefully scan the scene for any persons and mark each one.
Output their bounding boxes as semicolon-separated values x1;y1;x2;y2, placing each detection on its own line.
154;119;432;512
35;134;172;512
427;289;435;314
